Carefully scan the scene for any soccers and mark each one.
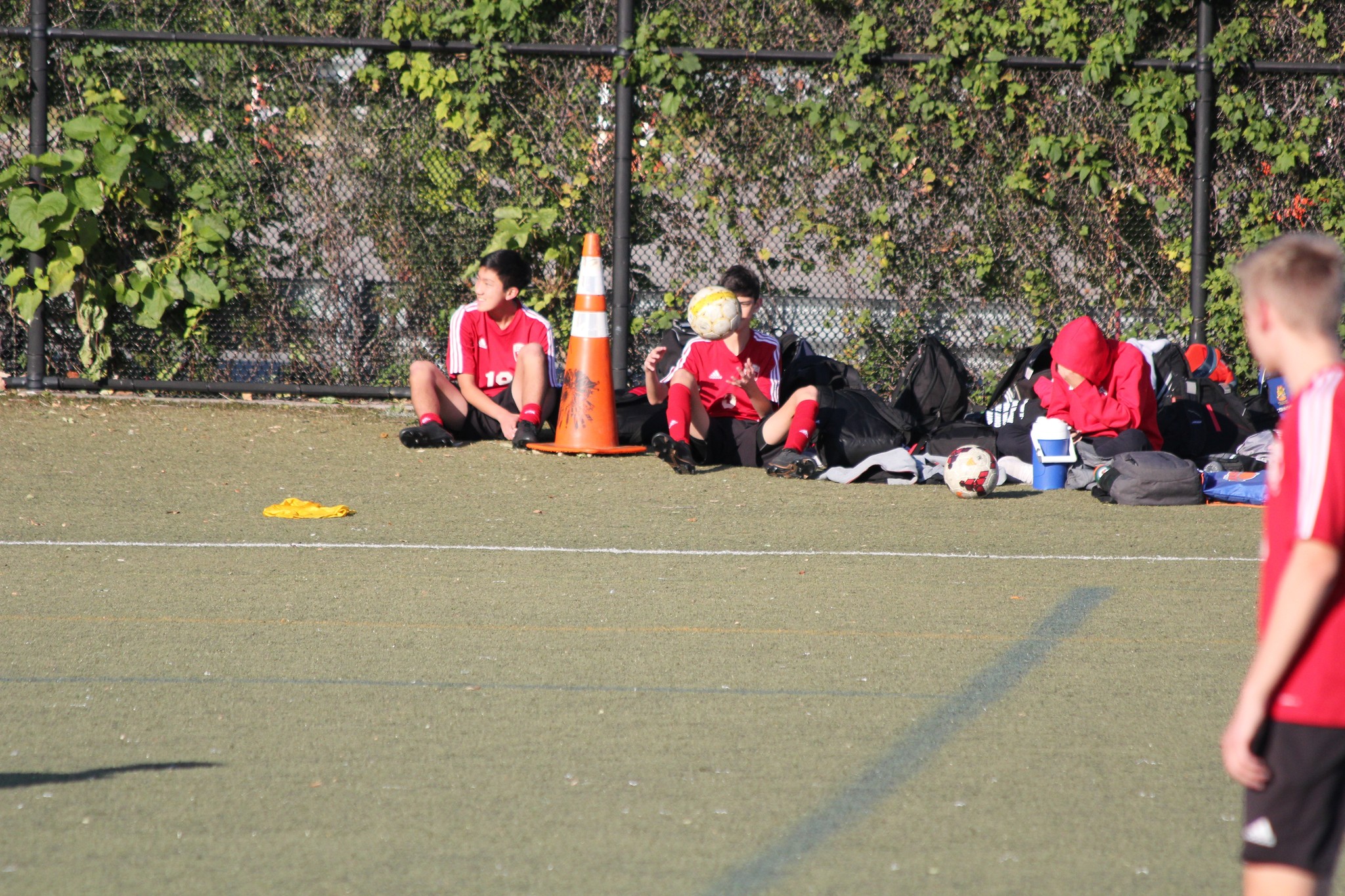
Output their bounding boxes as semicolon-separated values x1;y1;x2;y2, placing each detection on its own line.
944;445;999;500
688;286;740;341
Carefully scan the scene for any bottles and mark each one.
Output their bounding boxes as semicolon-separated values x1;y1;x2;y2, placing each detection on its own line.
1094;465;1120;491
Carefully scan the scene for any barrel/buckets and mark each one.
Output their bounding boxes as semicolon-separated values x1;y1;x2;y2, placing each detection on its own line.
1030;416;1077;491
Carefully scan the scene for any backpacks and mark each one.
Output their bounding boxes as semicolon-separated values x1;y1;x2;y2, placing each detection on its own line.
1091;342;1291;507
615;329;1051;466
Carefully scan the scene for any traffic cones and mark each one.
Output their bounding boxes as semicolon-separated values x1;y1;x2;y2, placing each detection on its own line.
523;227;653;456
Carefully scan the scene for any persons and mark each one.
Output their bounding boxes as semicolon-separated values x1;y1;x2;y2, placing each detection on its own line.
1033;315;1164;458
644;264;821;480
398;249;562;451
1220;230;1345;896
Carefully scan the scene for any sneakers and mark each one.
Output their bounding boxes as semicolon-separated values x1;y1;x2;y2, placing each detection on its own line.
512;419;540;448
767;452;817;479
399;420;455;448
651;433;697;475
